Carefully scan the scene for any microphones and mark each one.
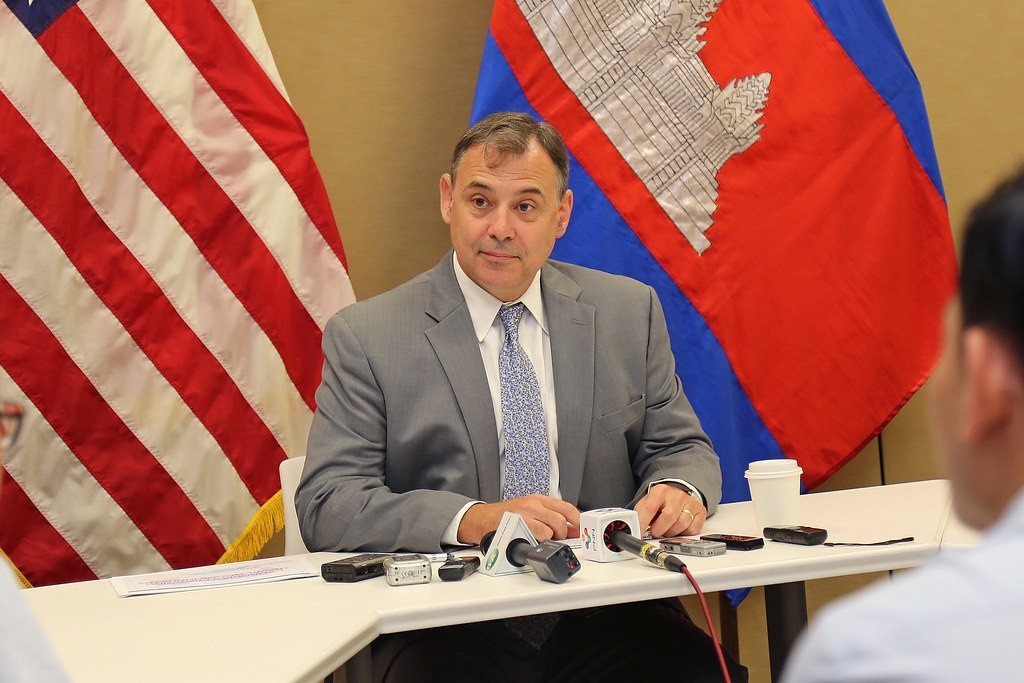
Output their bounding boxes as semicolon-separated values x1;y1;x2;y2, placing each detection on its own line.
580;507;687;573
481;529;582;584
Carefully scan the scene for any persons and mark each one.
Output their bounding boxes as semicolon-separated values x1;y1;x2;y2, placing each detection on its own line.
776;175;1024;683
294;111;748;683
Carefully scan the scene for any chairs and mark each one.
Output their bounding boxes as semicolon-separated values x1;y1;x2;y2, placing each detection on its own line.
279;454;310;557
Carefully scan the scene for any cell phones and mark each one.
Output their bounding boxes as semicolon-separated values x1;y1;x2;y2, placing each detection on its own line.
320;554;396;582
438;556;481;581
659;537;726;556
383;555;432;585
763;524;828;546
700;534;764;551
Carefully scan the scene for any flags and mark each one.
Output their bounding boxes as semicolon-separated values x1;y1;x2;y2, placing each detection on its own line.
469;1;958;606
0;0;359;586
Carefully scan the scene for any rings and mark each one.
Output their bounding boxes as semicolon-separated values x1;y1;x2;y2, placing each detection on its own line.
683;510;693;520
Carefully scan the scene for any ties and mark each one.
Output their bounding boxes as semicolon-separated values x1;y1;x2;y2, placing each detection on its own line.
497;304;550;501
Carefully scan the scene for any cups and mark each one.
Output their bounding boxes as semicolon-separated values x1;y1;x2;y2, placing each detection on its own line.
744;458;804;529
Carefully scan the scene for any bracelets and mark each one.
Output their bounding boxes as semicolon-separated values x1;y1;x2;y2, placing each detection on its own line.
668;482;701;504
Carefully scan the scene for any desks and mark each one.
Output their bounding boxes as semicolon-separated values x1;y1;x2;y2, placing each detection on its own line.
11;478;986;683
